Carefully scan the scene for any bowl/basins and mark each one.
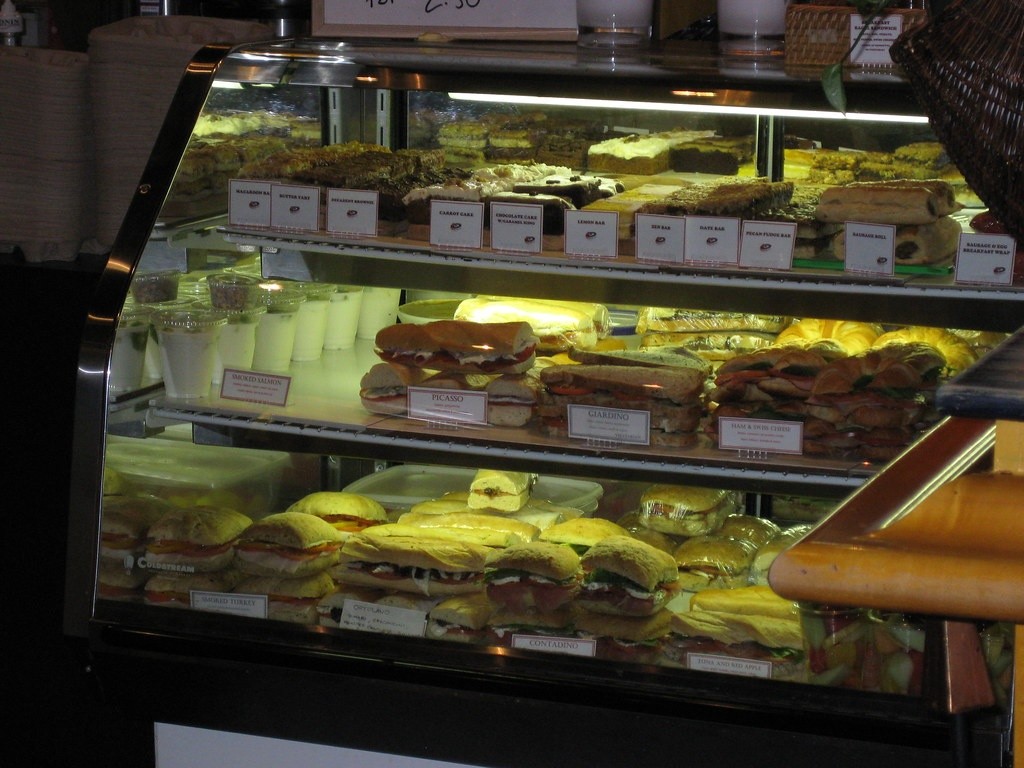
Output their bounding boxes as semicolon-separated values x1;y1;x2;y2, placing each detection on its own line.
395;297;462;325
204;273;262;310
132;270;180;302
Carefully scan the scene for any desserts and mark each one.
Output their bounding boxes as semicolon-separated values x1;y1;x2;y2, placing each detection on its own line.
154;115;305;200
230;140;846;263
437;110;984;207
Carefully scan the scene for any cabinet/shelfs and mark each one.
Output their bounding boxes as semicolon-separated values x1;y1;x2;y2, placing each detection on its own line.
63;34;1024;768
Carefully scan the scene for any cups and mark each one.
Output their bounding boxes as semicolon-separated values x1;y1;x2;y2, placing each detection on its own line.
719;0;787;59
575;0;654;53
153;268;400;403
97;260;256;401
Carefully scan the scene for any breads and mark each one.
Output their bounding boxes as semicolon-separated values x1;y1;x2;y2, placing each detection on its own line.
813;179;961;265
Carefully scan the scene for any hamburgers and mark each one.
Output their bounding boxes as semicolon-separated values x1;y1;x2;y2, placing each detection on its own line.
360;292;1007;464
99;466;834;652
